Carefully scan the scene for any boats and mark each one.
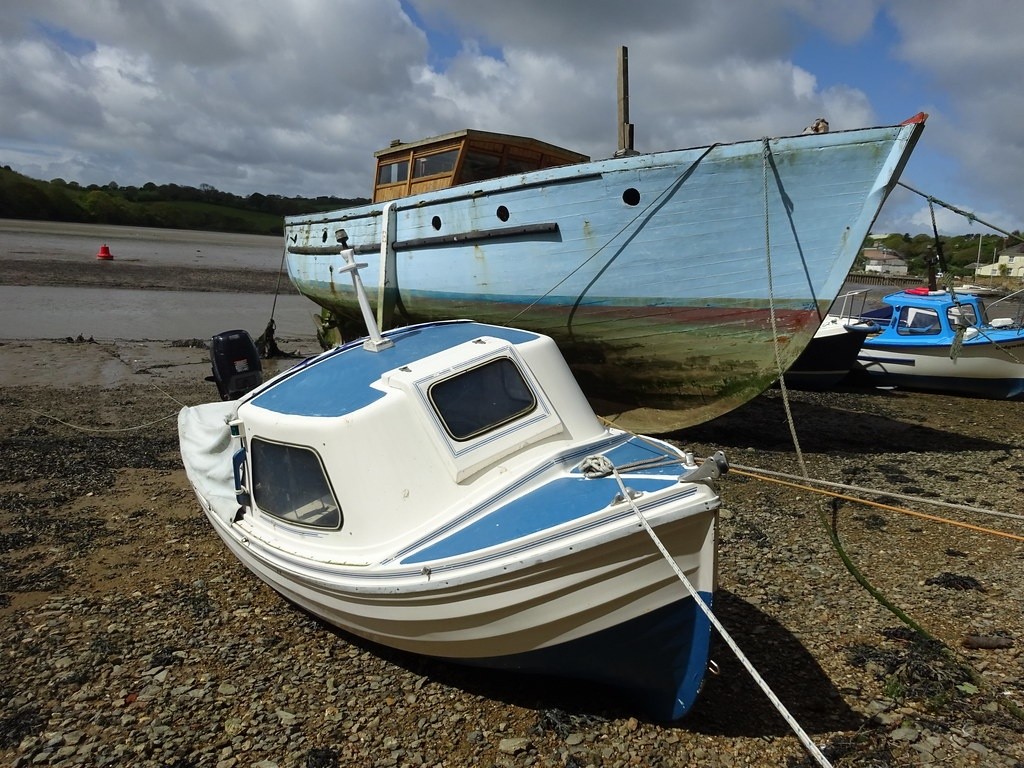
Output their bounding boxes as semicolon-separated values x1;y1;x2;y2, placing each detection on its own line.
176;319;727;662
279;46;928;435
855;277;1024;400
782;288;879;390
